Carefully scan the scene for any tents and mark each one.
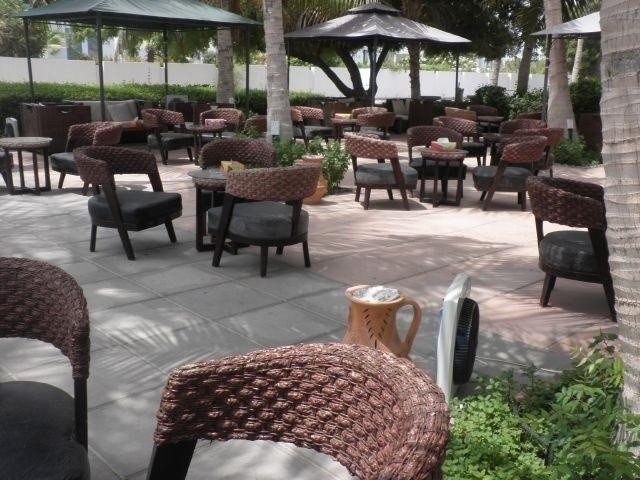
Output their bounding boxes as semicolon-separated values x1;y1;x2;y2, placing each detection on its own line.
282;0;473;100
529;12;602;35
6;0;263;122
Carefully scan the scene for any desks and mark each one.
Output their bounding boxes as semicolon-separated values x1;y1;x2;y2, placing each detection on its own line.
417;147;469;205
187;124;223;165
187;168;250;252
478;131;513;166
330;118;358;139
0;136;54;192
479;116;504;134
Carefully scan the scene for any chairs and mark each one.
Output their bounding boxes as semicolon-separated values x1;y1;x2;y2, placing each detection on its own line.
200;138;277;236
472;136;545;211
290;106;328;150
500;128;563;178
344;134;417;210
141;109;193;165
358;112;397;140
1;257;90;479
147;342;451;480
52;122;122;195
353;107;388;132
524;175;617;322
197;108;243;147
406;125;464;199
495;119;548;165
444;107;478;142
243;114;268;139
73;144;182;261
211;164;321;277
467;105;497;133
434;116;480;163
0;152;13;193
